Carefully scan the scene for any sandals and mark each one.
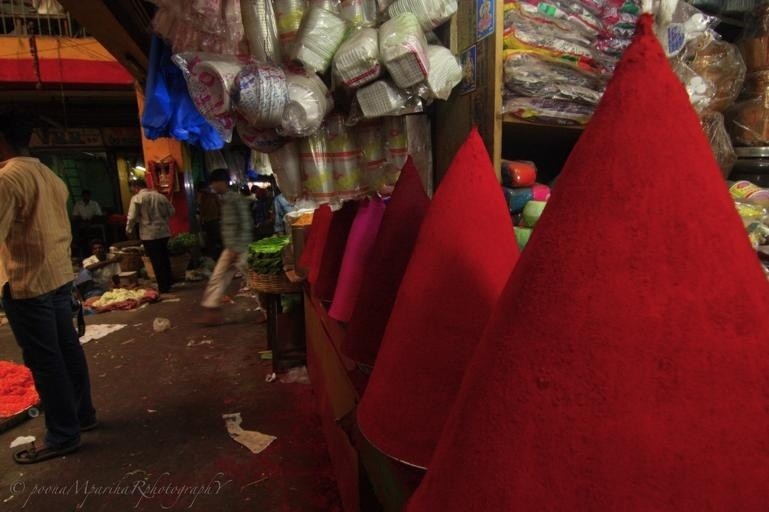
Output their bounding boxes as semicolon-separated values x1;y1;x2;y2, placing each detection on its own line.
13;436;82;463
78;407;98;432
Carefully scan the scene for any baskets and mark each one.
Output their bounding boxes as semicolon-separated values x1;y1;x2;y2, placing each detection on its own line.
246;268;303;294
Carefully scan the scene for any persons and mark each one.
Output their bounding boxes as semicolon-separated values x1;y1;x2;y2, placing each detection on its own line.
71;187;101;230
200;168;253;309
0;90;102;463
195;171;294;255
124;172;176;294
70;242;122;302
